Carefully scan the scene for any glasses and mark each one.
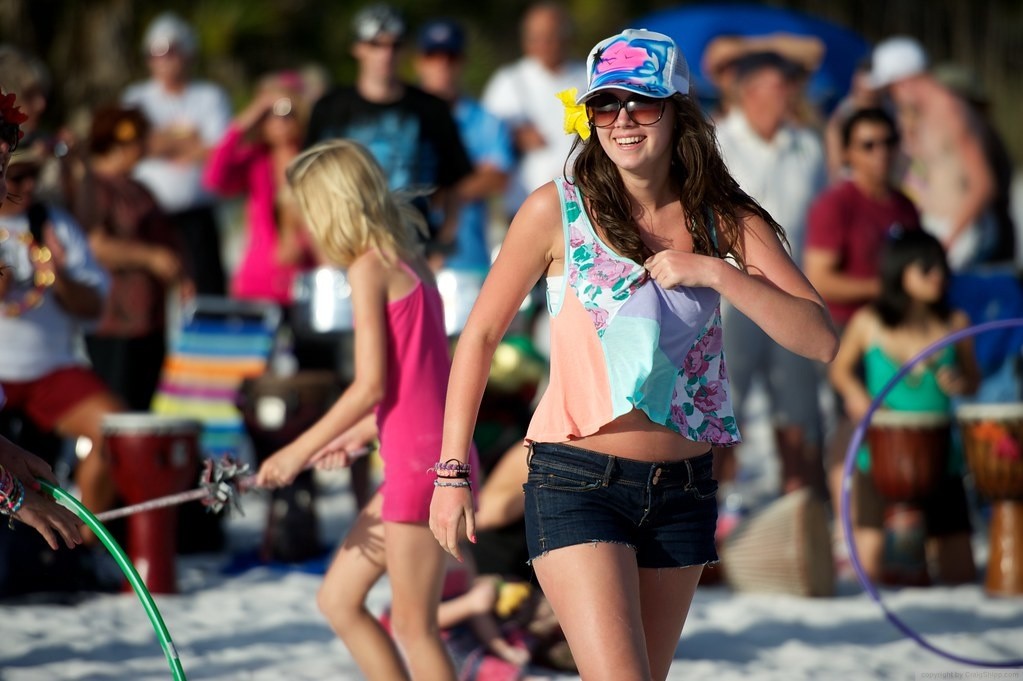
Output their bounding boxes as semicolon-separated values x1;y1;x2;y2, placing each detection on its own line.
910;252;943;272
858;137;896;151
585;92;670;127
5;165;41;185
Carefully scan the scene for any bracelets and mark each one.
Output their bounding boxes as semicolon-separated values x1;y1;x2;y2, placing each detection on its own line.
434;462;473;488
0;465;24;528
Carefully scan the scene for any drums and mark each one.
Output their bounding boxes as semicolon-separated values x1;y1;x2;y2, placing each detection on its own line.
869;411;951;585
102;415;202;594
699;485;834;600
235;369;336;564
957;402;1023;595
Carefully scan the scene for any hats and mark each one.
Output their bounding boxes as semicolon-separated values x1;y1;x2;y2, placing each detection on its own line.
351;7;406;48
87;106;149;155
576;28;690;105
865;35;926;91
421;21;468;64
144;16;198;57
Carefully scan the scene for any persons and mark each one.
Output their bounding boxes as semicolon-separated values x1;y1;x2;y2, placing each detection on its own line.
79;18;337;574
257;141;477;681
0;435;83;550
429;29;839;680
0;154;124;594
417;6;595;396
302;3;468;508
704;34;997;584
